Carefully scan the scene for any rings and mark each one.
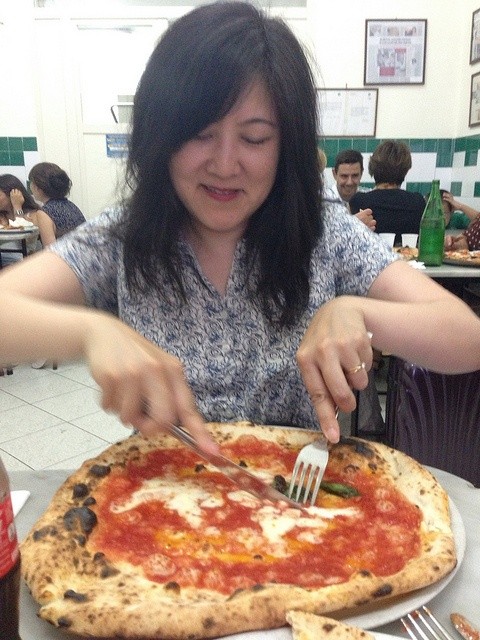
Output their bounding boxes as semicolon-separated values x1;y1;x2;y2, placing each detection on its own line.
345;361;366;375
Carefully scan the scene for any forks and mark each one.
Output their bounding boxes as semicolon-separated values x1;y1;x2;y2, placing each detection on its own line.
288;330;373;507
398;605;454;639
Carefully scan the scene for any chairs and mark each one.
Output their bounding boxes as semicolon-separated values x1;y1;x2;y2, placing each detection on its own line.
0;235;58;377
351;284;480;486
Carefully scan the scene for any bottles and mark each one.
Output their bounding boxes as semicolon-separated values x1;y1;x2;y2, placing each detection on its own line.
0;455;22;640
417;180;445;266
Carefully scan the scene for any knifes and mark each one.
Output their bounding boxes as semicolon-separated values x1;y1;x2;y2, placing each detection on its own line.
137;394;306;512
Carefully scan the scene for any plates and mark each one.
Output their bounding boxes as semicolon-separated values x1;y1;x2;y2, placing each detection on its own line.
223;491;467;636
0;228;39;233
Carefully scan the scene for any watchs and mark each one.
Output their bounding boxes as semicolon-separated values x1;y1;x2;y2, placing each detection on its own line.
12;209;24;216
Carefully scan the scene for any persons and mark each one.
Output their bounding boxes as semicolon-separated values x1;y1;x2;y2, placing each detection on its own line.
0;1;480;457
426;188;480;231
0;173;41;229
331;148;372;206
442;208;480;252
317;148;377;232
28;162;86;251
348;140;427;248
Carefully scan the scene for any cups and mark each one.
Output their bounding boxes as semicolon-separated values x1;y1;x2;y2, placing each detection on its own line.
401;233;419;249
378;233;396;249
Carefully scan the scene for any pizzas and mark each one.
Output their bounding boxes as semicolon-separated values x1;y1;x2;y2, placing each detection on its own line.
445;250;480;263
25;421;457;636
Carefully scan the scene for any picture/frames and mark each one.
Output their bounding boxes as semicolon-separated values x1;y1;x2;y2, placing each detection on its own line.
468;72;480;129
468;6;480;66
315;86;381;138
363;18;428;87
